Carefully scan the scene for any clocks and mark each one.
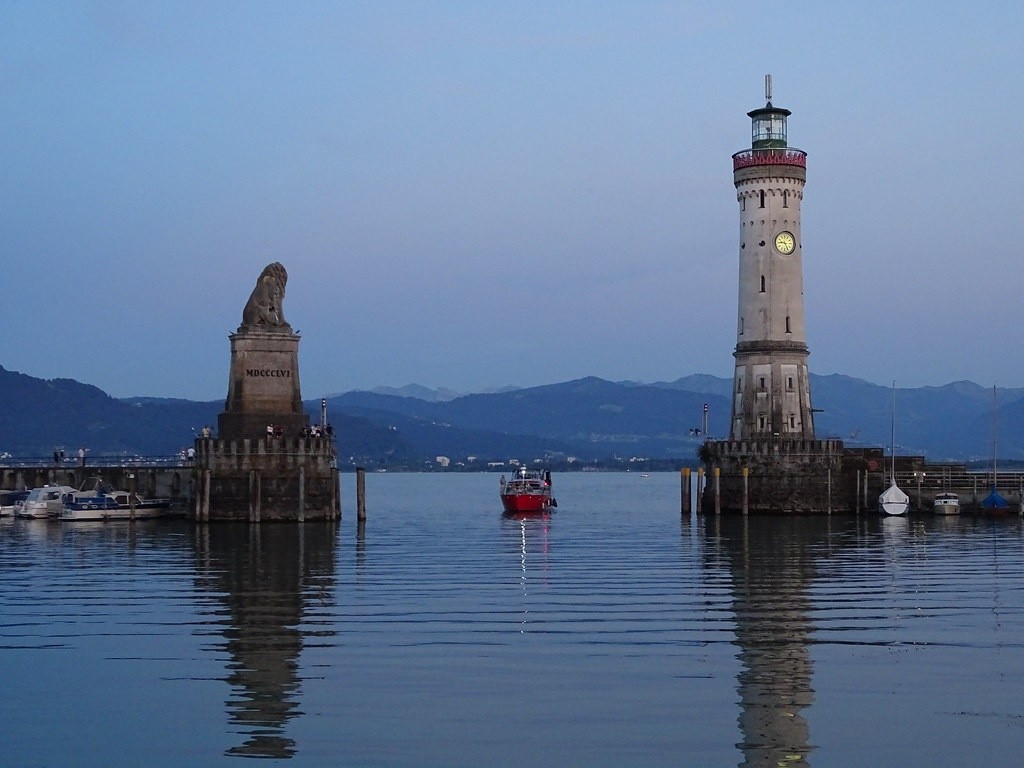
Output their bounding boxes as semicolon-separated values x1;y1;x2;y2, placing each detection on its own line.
776;232;794;253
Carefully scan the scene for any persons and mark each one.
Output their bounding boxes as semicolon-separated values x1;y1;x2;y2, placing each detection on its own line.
267;423;274;448
54;447;86;466
274;425;283;438
187;447;195;460
500;475;507;495
300;423;332;448
202;425;210;438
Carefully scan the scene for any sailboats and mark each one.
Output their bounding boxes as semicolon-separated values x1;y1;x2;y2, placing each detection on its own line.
878;378;910;517
975;384;1011;509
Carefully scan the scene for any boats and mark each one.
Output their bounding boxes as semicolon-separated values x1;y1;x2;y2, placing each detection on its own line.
499;464;558;510
933;492;962;516
1;477;171;521
